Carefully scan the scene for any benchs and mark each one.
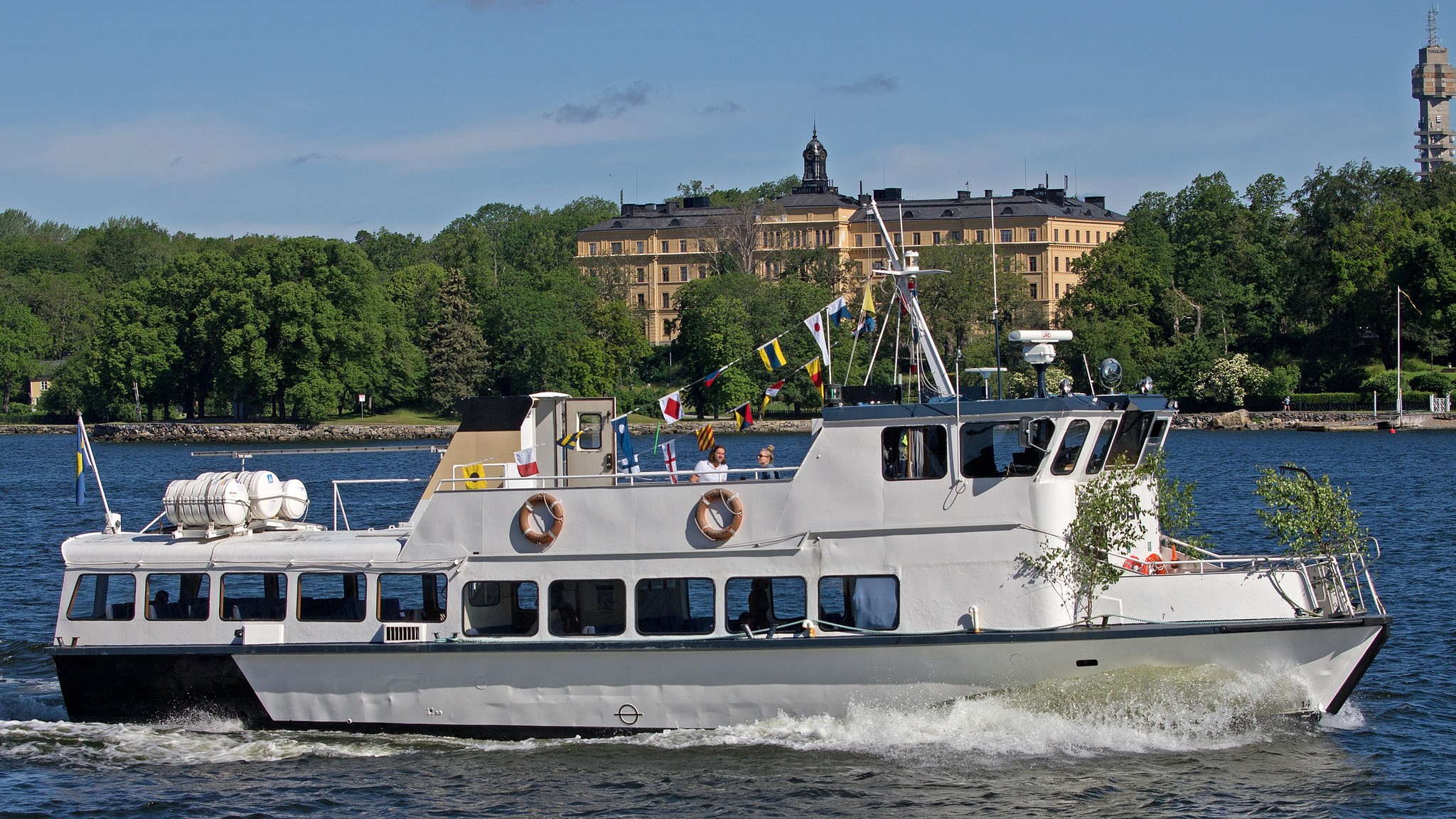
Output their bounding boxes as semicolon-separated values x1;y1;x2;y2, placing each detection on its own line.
106;598;406;622
467;617;729;634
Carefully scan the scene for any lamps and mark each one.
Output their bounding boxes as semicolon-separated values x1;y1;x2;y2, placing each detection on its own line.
1166;399;1178;409
1109;401;1119;410
1059;378;1072;396
1098;358;1123;394
823;384;842;407
1135;376;1153;395
903;250;920;271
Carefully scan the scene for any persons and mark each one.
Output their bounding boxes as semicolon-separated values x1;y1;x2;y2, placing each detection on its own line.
527;587;562;635
1282;396;1290;411
757;445;781;480
748;589;783;633
689;444;728;482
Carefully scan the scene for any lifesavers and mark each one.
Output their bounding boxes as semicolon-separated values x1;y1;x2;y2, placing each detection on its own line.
518;492;563;545
695;486;742;541
1141;553;1165;576
1123;555;1142;571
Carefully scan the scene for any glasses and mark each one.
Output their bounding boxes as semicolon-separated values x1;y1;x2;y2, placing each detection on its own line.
757;454;768;458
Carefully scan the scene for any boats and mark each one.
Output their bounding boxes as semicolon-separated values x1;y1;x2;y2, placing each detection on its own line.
53;184;1393;751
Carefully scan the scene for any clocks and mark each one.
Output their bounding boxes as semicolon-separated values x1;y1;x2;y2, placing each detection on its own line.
820;150;826;160
805;150;815;160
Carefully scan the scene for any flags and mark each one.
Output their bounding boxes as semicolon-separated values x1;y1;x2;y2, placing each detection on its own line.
695;422;715;453
76;421;94;507
661;439;678;484
863;283;876;320
756;338;787;374
762;378;784;417
805;356;824;398
658;390;686;425
803;311;830;366
621;454;640;479
705;364;728;389
734;401;754;430
462;460;488;489
556;429;583;450
610;413;635;466
513;446;540;477
